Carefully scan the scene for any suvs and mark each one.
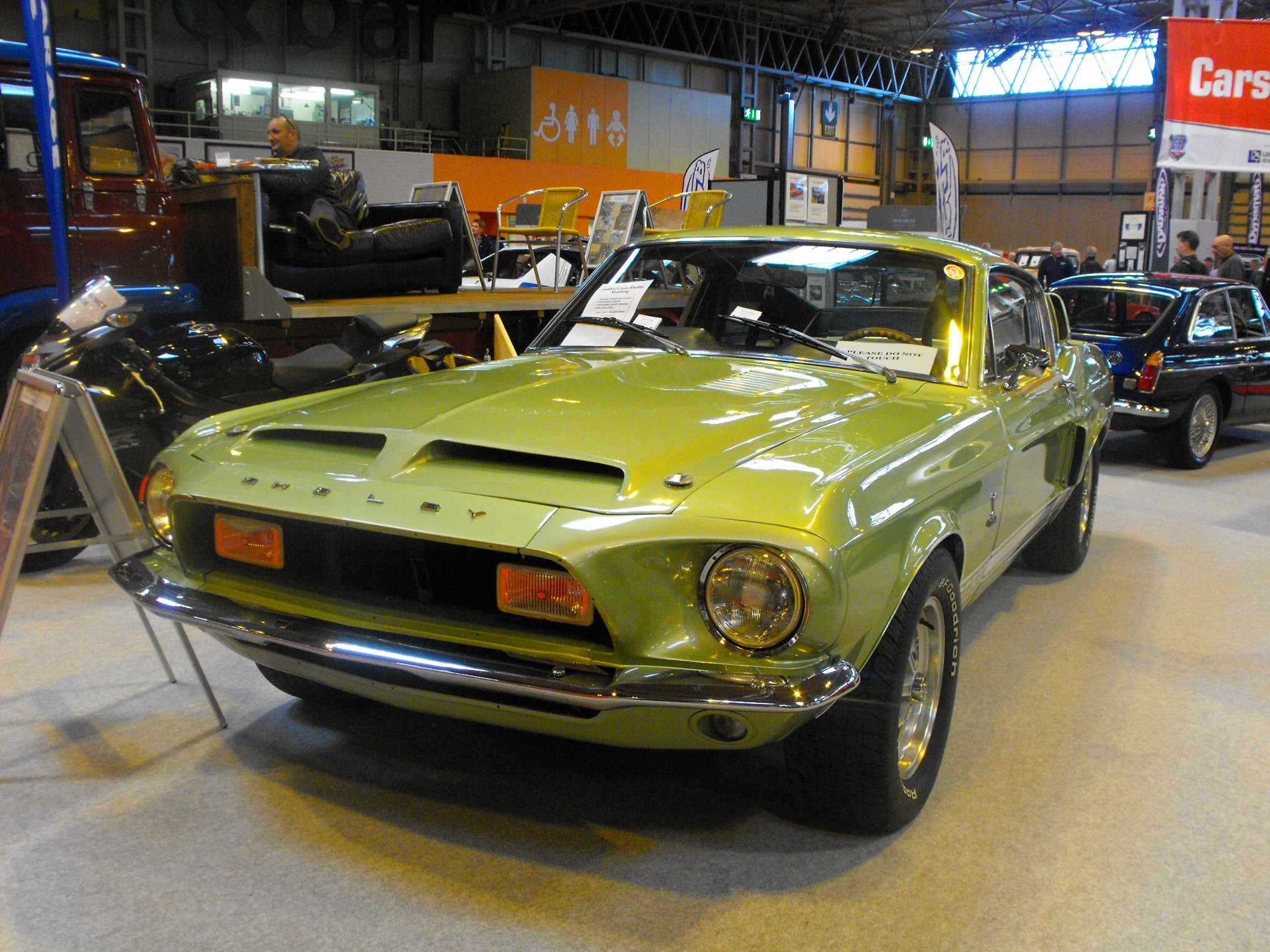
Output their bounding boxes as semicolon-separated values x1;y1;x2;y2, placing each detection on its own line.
1013;245;1081;290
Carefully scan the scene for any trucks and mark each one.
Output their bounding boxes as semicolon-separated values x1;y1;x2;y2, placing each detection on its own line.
0;54;182;325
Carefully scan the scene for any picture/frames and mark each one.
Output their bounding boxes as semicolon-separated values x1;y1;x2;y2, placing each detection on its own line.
320;149;356;170
204;140;276;166
157;139;187;160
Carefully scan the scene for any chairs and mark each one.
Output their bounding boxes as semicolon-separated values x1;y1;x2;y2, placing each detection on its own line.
492;187;591;294
639;189;733;291
702;280;787;352
81;125;144;176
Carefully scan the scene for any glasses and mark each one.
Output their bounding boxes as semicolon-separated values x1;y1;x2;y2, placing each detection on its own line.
271;114;296;130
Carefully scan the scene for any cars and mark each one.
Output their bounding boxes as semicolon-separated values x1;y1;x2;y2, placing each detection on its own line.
117;229;1116;845
456;244;589;286
1043;272;1270;469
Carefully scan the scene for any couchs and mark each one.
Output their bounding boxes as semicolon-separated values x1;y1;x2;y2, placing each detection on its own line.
256;167;464;298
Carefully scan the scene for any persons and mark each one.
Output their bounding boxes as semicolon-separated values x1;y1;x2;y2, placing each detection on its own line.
983;230;1270;311
471;219;496;260
268;114;356;250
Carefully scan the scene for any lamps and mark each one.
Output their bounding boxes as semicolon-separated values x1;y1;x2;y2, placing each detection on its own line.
1075;21;1091;37
1092;19;1107;37
921;41;934;52
909;44;923;54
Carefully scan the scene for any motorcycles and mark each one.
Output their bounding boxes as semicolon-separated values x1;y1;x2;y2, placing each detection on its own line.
15;267;456;578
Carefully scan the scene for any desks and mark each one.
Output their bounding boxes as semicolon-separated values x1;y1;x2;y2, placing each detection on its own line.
196;163;315;302
168;175;257;303
